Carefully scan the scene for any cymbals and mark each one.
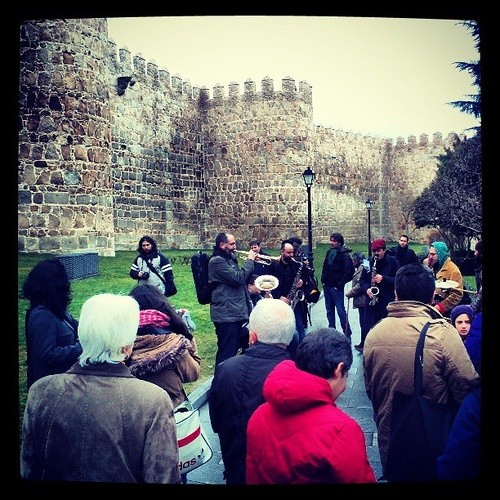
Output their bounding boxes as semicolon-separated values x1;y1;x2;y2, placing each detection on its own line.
437;278;459;289
255;275;279;290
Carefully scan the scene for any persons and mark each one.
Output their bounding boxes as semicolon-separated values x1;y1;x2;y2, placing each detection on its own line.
359;259;484;484
246;326;377;484
206;295;299;484
119;283;203;412
196;228;486;383
20;252;86;380
129;235;181;300
19;291;185;486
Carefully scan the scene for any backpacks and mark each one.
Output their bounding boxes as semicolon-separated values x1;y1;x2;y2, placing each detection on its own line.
301;261;321;303
191;249;232;305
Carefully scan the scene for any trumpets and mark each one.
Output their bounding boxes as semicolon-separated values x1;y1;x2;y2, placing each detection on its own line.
232;249;282;265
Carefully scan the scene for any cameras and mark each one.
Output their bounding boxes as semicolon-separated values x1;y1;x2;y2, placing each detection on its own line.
139;272;149;280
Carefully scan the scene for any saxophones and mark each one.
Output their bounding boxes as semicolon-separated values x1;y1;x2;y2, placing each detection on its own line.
285;257;306;311
369;255;379;307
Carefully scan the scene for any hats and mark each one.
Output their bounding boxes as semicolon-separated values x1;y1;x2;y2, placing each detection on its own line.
450;305;475;323
475;240;483;252
370;239;386;250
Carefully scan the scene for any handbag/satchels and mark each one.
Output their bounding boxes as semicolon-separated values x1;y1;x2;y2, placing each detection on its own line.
174;399;214;475
385;322;463;483
165;279;178;298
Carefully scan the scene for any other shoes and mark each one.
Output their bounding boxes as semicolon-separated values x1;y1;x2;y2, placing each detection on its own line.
355;344;363;353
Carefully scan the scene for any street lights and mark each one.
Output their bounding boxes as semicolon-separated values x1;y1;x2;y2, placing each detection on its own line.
301;166;319;286
364;198;373;260
